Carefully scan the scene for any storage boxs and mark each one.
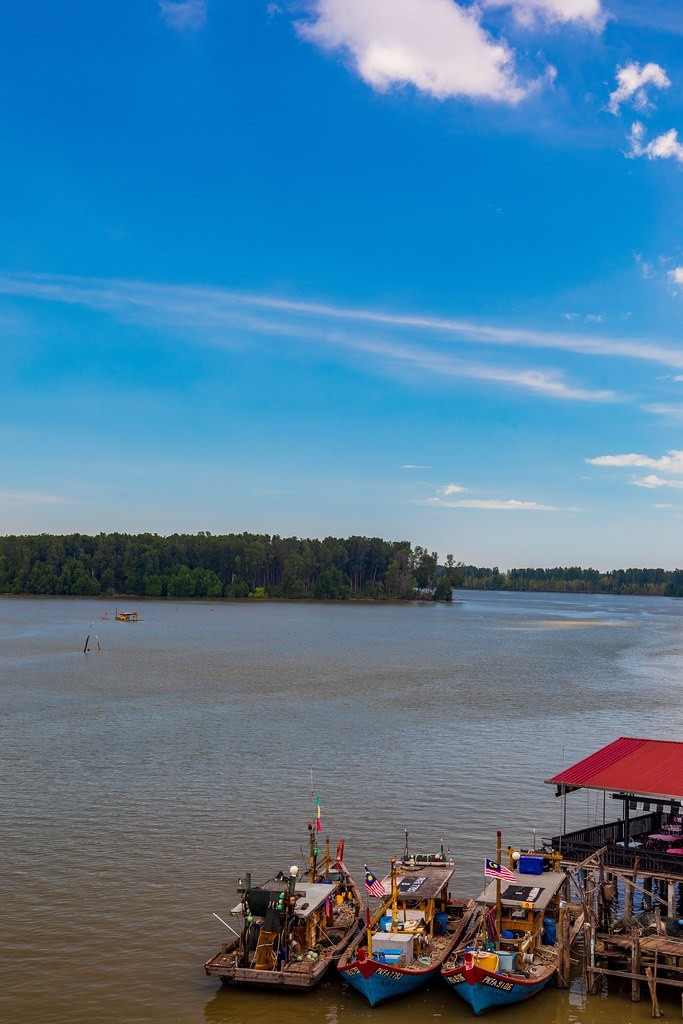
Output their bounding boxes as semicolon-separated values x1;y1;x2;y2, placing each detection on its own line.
470;952;500;974
372;932;414;965
494;951;519;972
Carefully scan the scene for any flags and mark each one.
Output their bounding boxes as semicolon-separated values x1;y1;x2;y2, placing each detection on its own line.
364;865;386;900
484;856;517;884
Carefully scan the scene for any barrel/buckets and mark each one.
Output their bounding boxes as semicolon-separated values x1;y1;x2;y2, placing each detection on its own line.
434;912;447;935
542;918;556;945
380;916;392;930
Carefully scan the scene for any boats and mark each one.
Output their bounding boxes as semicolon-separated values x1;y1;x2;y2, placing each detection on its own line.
439;829;587;1016
116;610;139;621
204;762;365;989
337;828;478;1009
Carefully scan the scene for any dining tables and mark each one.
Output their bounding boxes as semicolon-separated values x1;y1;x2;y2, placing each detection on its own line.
648;816;683;855
